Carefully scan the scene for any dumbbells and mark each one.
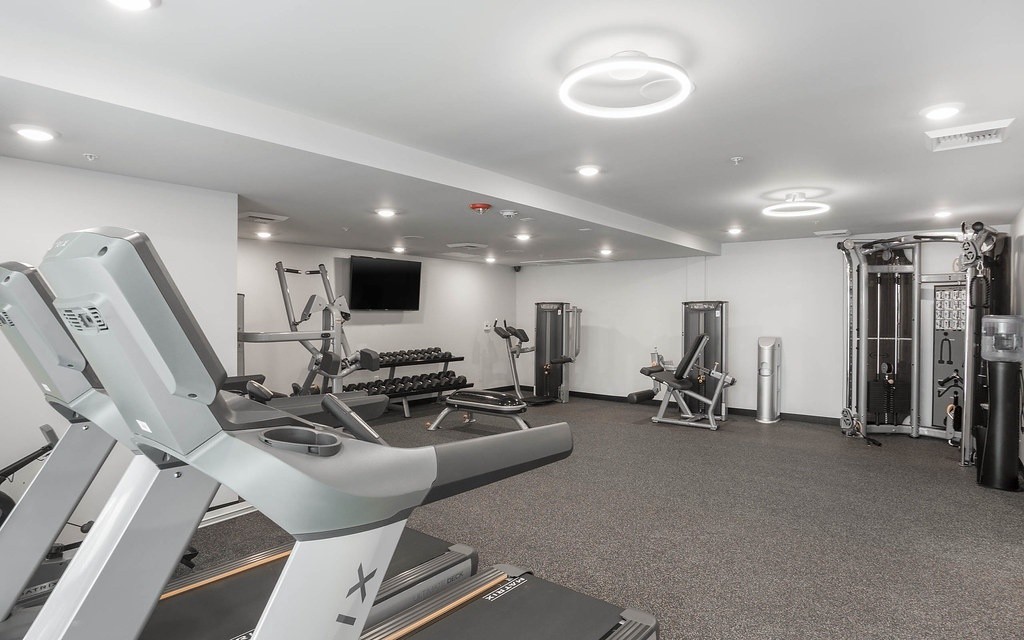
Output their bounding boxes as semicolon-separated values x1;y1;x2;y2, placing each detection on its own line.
327;346;468;397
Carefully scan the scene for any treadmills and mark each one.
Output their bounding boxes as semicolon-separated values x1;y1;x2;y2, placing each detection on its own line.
20;220;663;640
0;258;482;640
